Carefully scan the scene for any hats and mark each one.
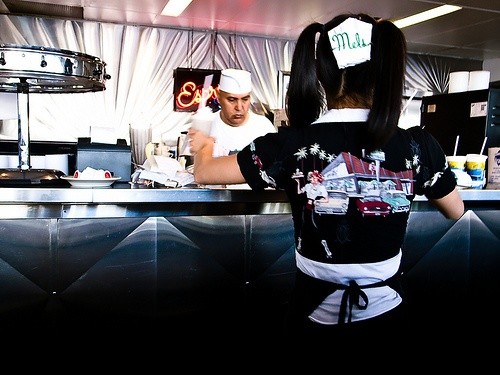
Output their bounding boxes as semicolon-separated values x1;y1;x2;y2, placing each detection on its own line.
219;69;251;94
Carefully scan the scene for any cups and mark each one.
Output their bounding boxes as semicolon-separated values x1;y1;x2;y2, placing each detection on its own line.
466;154;488;190
447;156;466;173
487;148;500;189
129;121;152;168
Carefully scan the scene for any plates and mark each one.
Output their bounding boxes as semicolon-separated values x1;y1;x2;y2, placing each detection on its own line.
61;176;121;187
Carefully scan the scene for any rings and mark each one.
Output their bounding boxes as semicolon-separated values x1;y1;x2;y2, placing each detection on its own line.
188;138;192;142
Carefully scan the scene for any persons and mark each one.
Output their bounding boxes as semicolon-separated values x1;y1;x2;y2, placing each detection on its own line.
186;12;464;375
178;68;279;155
